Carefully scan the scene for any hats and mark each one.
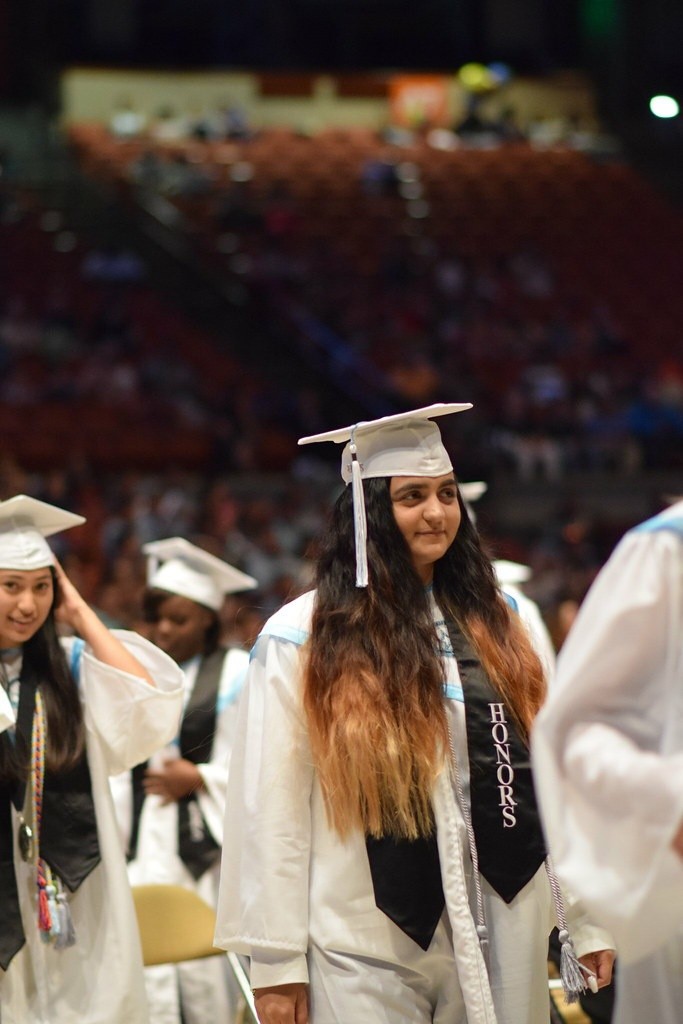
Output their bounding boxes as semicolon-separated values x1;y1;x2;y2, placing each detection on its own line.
491;559;532;582
297;402;473;588
0;494;87;571
457;481;487;524
142;536;257;611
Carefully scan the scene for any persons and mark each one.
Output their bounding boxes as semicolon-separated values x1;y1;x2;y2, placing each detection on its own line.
0;96;683;1024
213;402;616;1024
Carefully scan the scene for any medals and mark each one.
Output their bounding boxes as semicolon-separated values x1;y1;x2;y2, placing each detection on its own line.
18;823;33;862
